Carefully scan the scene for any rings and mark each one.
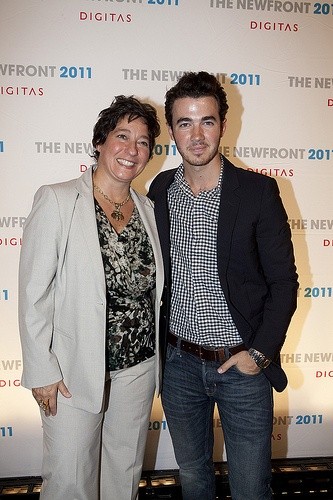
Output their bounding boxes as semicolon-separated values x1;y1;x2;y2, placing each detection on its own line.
38;400;49;411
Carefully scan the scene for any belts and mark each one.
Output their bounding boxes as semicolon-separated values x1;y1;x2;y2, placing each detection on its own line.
166;331;247;362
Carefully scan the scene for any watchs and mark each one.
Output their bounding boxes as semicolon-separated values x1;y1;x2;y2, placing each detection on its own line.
248;348;272;369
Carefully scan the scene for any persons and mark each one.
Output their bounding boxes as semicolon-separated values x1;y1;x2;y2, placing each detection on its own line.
18;95;165;500
146;72;298;500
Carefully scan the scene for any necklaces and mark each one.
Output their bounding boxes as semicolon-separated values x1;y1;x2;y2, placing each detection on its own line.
93;182;131;222
